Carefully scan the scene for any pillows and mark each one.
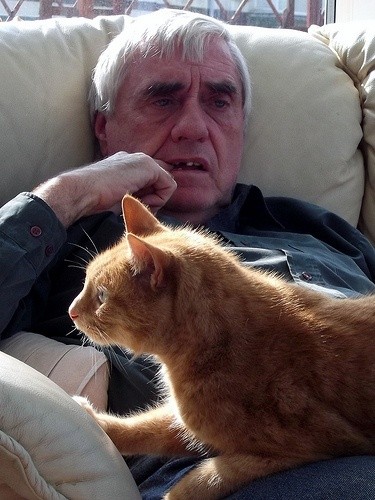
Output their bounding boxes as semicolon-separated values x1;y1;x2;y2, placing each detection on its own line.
1;334;105;423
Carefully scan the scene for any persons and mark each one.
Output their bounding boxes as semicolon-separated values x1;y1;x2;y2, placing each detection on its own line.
0;8;375;500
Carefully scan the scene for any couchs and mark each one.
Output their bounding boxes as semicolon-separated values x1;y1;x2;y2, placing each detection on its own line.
1;16;375;500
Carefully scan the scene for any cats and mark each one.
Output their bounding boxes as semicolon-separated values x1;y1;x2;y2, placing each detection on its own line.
67;191;375;500
